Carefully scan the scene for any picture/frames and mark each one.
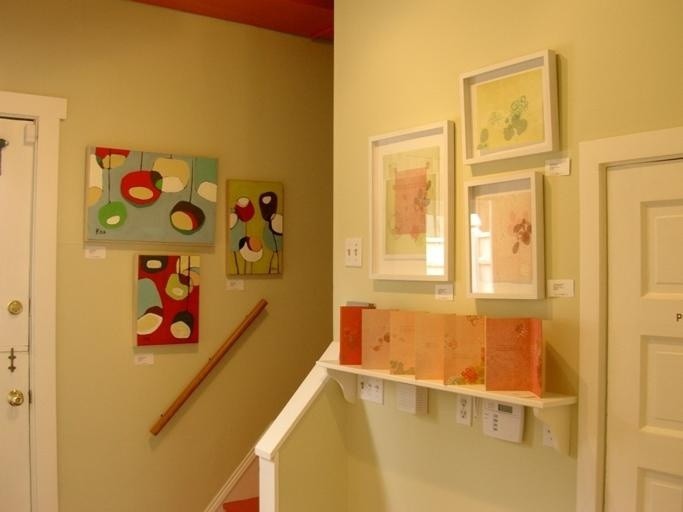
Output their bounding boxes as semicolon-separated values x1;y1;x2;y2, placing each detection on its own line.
366;122;458;284
459;50;560;163
464;171;546;303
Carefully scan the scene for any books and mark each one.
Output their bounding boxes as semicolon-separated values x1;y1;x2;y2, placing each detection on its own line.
483;316;543;397
412;312;455;380
359;308;400;369
339;306;370;365
442;315;483;386
387;309;435;375
345;301;371;309
